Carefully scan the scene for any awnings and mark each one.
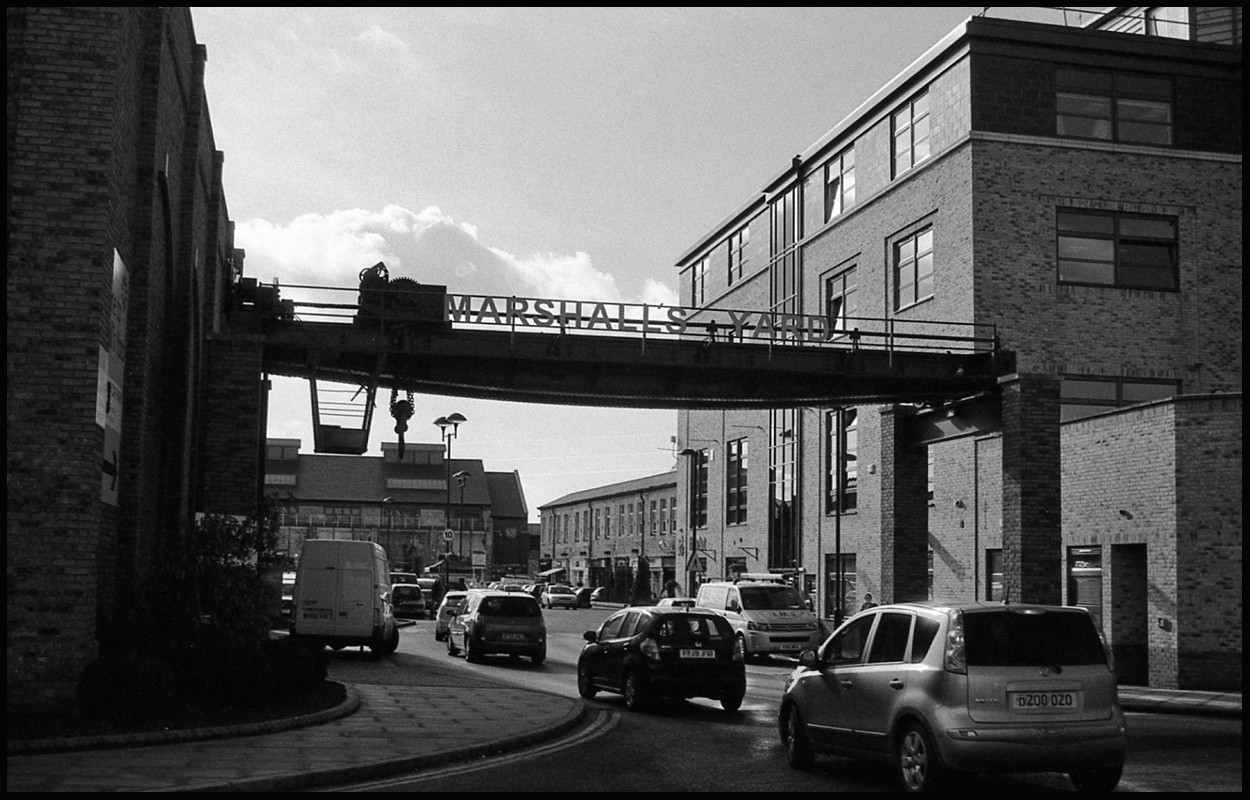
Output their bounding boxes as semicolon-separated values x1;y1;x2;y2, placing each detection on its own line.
537;568;564;576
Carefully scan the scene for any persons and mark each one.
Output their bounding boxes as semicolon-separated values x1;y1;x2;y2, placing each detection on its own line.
459;578;467;591
728;564;749;584
862;594;878;611
429;579;441;620
660;576;676;598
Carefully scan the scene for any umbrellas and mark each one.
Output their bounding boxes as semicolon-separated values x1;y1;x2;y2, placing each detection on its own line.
424;554;473;586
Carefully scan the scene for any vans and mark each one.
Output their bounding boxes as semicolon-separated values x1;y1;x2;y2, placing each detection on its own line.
291;540;399;656
700;578;820;663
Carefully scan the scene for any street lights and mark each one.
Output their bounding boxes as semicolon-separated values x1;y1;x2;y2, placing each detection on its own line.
433;413;466;593
382;497;398;559
677;448;697;596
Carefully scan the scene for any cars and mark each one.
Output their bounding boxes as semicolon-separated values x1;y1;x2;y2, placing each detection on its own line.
541;586;577;610
446;591;547;663
435;592;466;641
577;607;748;712
779;599;1127;800
488;581;605;609
656;598;697;607
390;572;439;620
